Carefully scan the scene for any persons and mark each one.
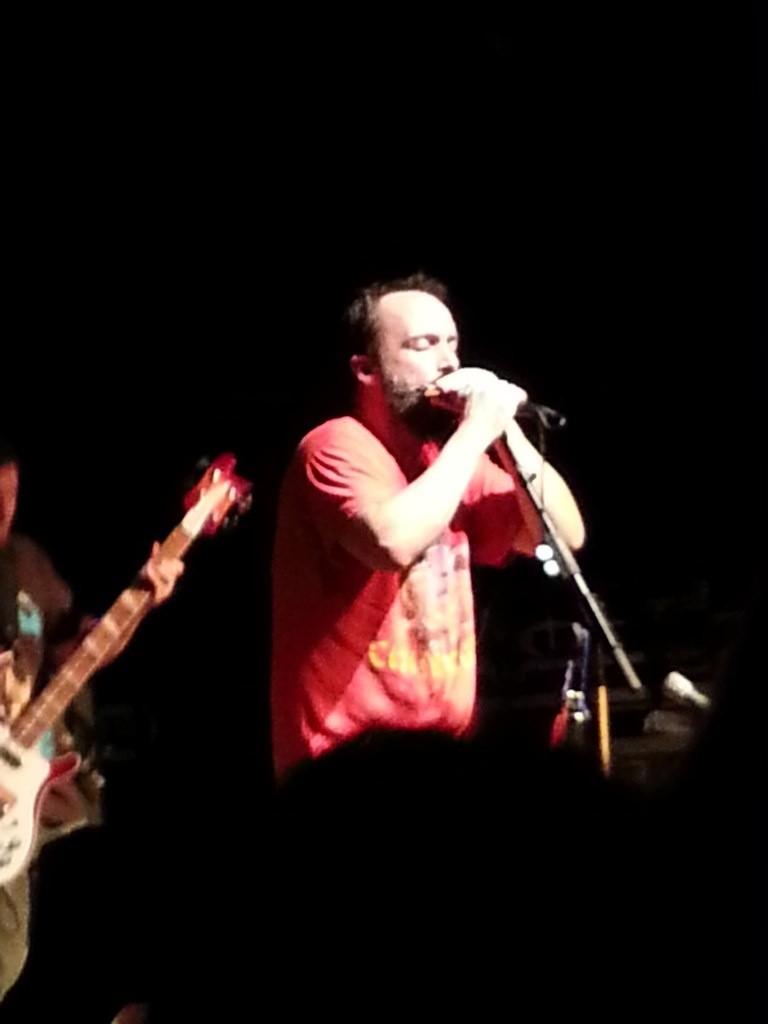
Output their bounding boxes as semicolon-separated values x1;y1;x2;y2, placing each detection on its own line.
264;265;597;1024
0;449;113;1022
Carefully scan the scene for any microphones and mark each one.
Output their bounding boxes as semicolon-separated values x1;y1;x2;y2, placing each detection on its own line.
514;400;567;429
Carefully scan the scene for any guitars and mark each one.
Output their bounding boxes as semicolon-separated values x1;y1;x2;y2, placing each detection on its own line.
0;449;254;884
